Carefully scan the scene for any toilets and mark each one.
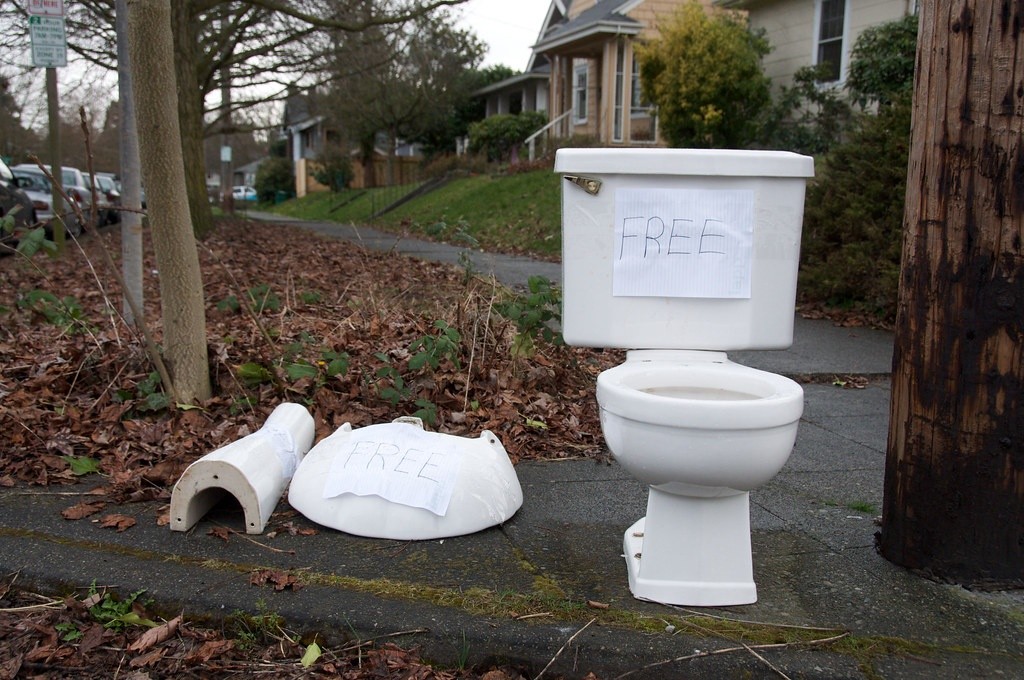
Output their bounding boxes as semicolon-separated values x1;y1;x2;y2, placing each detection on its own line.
552;148;816;606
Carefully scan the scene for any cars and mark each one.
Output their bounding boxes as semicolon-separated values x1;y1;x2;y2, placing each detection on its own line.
0;159;145;236
220;184;256;200
